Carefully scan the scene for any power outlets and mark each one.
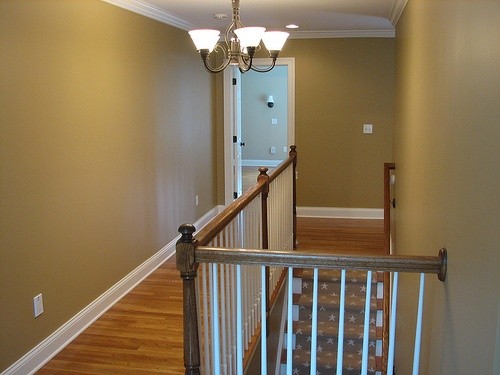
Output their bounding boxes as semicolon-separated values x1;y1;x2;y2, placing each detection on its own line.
271;147;275;153
34;294;44;317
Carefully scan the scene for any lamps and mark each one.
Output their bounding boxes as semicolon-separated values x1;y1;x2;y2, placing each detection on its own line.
188;0;290;74
